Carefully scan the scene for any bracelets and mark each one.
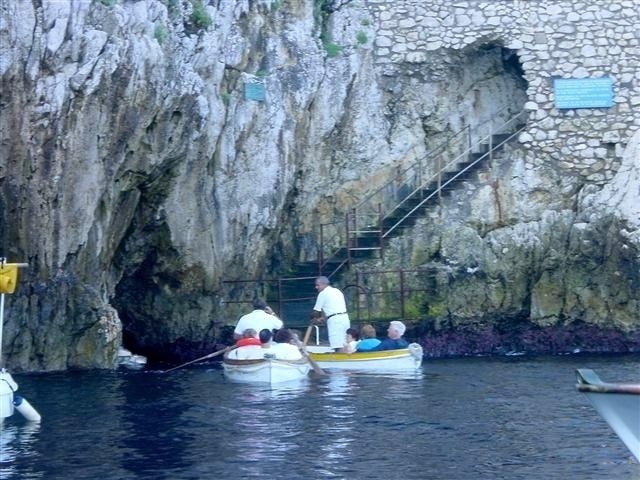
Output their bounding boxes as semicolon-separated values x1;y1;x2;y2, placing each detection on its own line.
271;312;275;316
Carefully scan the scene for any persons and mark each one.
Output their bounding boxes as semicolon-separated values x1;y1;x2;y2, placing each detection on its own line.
354;320;410;353
231;297;284;340
257;328;275;349
225;328;262;352
334;328;359;353
289;328;303;346
308;275;350;352
355;323;382;352
273;328;292;343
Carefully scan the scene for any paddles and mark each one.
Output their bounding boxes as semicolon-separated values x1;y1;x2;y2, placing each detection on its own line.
164;345;235;372
289;329;326;375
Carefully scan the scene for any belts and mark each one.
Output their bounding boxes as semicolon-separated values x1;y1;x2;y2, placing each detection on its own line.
327;311;347;320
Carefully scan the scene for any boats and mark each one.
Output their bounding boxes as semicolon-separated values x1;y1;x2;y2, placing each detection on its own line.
221;346;311;380
301;339;426;375
575;369;640;464
118;348;148;372
0;257;42;424
